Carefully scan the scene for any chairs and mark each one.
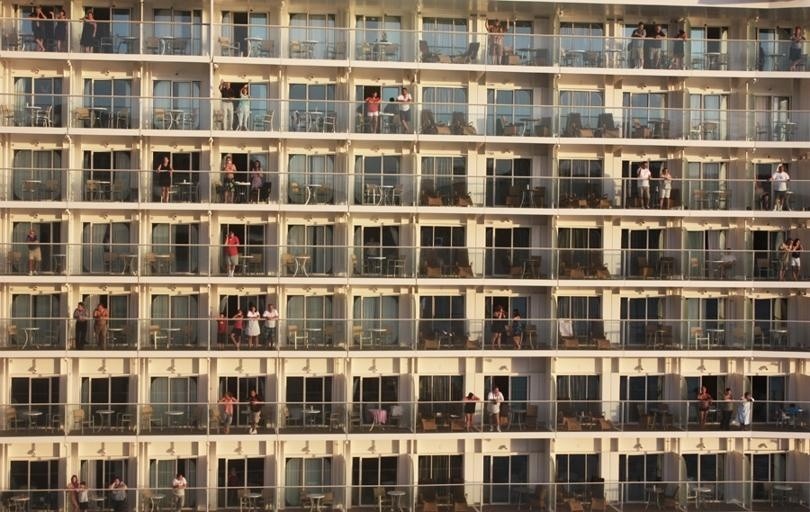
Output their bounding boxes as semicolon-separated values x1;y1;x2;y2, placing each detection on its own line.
1;32;808;71
17;179;796;210
9;324;792;352
3;487;809;511
4;406;808;434
2;102;797;140
9;249;789;279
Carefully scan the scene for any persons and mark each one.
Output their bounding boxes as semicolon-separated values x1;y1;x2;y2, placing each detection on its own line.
218;304;280;350
397;87;413;134
789;26;806;71
384;97;399;133
249;160;264;203
157;156;173;203
223;156;236;203
769;166;790;211
631;22;687;69
65;475;90;512
225;231;241;277
25;231;42;275
696;386;754;431
246;389;265;432
487;385;505;432
720;248;736;279
73;301;109;350
463;392;480;431
366;91;381;133
779;238;803;281
218;392;237;433
172;473;187;512
109;477;129;512
229;467;241;505
492;304;522;350
220;78;250;131
638;161;672;209
28;5;97;51
485;16;510;65
